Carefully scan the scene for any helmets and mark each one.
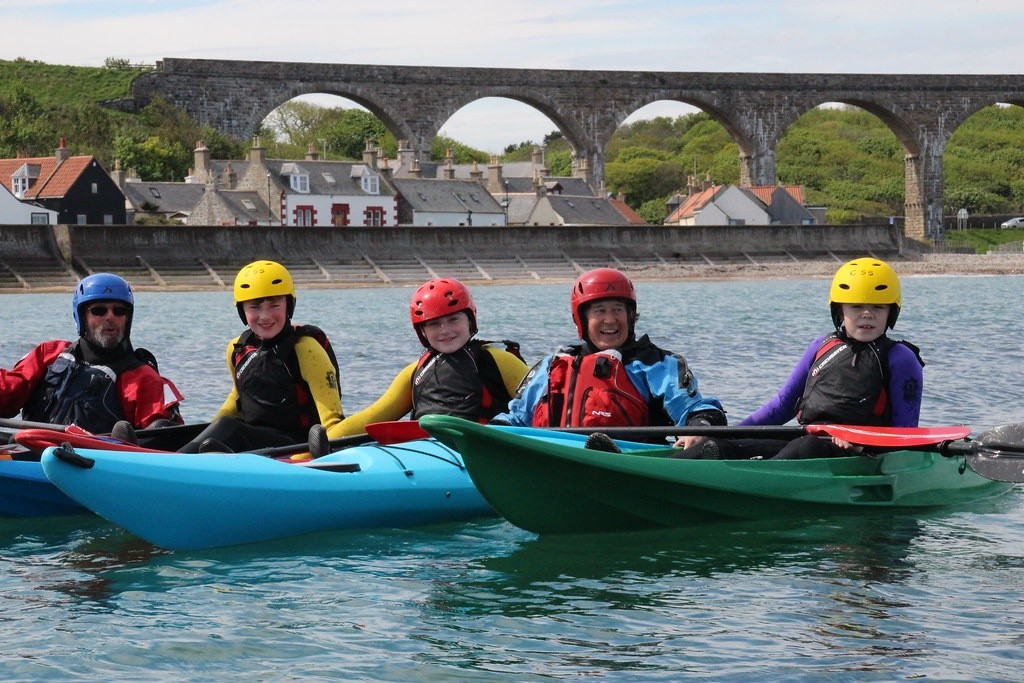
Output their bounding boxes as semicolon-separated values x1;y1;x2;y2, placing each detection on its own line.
570;268;637;341
829;257;902;331
234;260;296;325
410;277;479;348
72;273;135;337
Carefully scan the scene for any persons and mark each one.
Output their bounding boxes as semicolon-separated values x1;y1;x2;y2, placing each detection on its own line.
291;277;529;459
488;268;728;450
585;258;926;459
0;274;185;445
110;260;345;456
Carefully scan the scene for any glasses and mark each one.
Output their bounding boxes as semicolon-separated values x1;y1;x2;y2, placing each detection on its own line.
81;305;128;318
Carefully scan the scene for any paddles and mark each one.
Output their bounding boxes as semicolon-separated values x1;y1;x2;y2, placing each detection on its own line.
814;422;1024;484
361;419;975;450
249;431;373;459
0;416;143;449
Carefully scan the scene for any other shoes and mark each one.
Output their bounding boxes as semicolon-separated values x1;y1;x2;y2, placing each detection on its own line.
110;422;139;447
584;433;621;454
199;439;234;455
308;424;334;458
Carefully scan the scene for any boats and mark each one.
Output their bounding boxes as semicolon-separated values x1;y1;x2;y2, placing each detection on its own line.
0;420;212;519
418;414;1017;536
40;425;685;556
